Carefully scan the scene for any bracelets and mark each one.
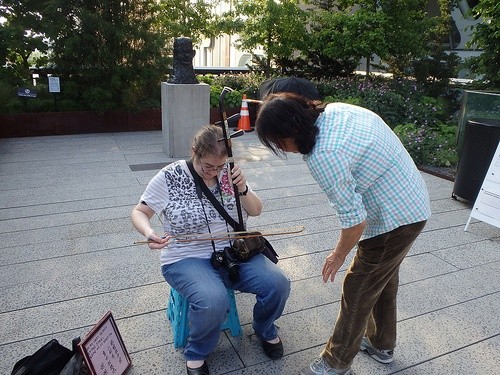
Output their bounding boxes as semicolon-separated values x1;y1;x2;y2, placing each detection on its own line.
239;185;248;196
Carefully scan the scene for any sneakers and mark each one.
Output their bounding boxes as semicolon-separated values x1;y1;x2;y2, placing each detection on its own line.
300;357;352;375
359;337;395;364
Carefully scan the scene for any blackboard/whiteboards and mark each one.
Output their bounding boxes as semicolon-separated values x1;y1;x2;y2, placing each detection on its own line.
470;141;500;228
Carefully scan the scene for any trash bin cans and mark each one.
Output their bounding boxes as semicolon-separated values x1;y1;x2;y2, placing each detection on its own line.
451;120;500;204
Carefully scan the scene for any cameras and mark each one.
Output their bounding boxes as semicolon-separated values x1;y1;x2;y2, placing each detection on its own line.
210;246;241;282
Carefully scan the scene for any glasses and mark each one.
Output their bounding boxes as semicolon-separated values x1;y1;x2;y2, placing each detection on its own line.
197;157;229;173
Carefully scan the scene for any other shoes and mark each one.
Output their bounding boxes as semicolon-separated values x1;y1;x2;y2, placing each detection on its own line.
257;335;284;360
186;360;210;375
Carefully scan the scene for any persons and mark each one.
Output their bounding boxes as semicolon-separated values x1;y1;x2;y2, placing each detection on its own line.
256;93;431;375
130;124;291;375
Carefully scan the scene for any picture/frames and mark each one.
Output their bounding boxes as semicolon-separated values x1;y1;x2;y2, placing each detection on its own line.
77;311;131;375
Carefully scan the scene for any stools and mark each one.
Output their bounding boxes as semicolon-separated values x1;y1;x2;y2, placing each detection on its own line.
167;285;243;350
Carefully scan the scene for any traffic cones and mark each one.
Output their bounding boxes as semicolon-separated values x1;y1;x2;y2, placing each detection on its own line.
233;94;255;132
